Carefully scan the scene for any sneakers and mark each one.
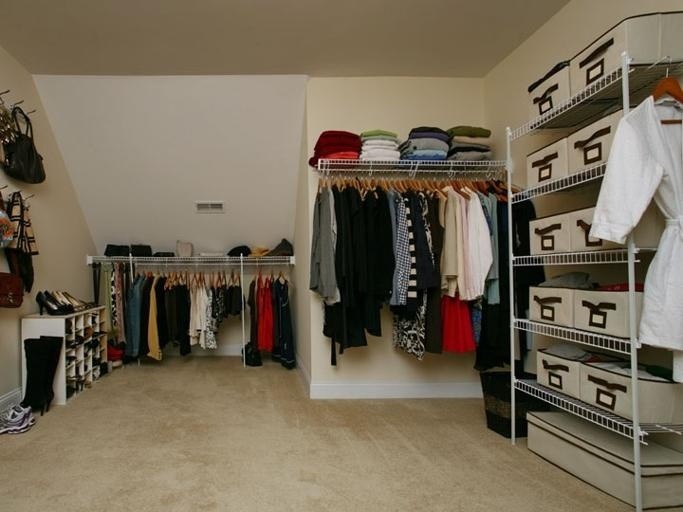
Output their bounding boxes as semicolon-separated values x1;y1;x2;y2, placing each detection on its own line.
0;406;35;433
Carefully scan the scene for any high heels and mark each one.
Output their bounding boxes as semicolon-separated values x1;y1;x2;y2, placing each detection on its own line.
36;286;93;315
65;314;108;398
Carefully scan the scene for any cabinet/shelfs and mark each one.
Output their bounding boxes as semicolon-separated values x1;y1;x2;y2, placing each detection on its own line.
504;52;680;512
20;304;108;406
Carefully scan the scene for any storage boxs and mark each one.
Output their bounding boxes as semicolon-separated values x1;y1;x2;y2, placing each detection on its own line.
535;347;627;401
568;108;639;187
527;138;567;192
526;411;682;510
572;198;666;252
528;60;620;135
528;285;575;329
573;287;647;339
528;211;571;257
568;10;683;105
580;365;683;424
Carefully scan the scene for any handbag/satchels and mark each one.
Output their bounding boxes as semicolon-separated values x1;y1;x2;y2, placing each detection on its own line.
2;106;45;183
0;273;23;307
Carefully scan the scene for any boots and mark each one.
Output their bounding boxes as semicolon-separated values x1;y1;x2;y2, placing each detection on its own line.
20;336;63;415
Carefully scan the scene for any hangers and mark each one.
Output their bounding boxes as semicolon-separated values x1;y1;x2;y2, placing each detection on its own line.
624;56;683;128
311;157;530;211
131;265;290;291
